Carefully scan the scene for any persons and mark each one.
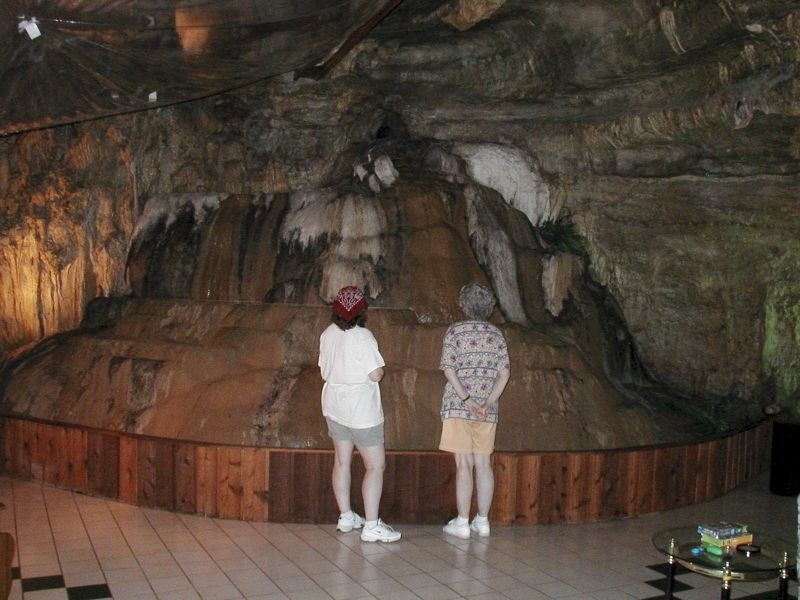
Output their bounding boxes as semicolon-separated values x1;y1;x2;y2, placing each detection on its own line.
318;286;401;542
439;282;510;538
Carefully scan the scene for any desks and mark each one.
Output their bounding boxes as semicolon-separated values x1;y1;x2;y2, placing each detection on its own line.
652;526;797;600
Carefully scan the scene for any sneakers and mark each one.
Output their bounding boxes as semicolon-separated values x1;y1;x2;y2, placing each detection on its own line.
470;513;491;538
336;513;366;532
361;517;401;543
443;518;470;539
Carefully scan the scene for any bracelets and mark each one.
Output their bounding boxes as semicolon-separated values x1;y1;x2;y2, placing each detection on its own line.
462;394;470;402
